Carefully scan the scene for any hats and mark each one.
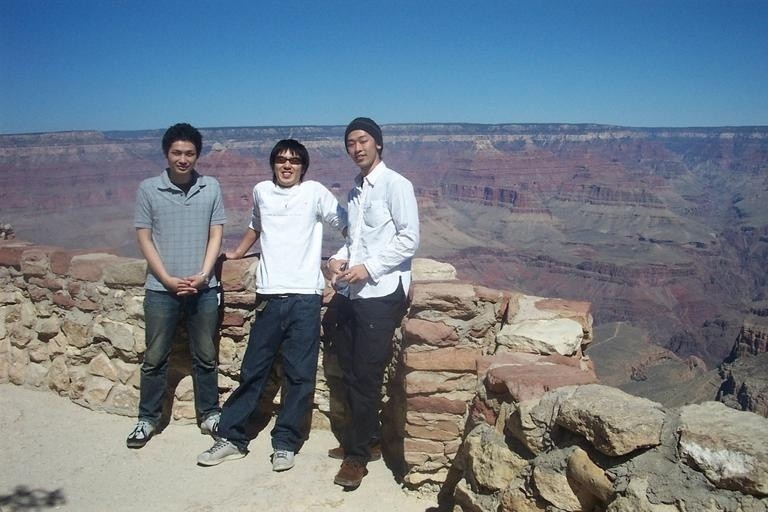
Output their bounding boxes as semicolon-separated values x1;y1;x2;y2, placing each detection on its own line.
344;117;383;155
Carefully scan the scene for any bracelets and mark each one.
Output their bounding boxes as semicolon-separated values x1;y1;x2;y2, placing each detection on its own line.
199;271;210;283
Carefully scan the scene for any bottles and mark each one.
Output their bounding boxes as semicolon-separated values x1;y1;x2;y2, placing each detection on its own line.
334;273;349;293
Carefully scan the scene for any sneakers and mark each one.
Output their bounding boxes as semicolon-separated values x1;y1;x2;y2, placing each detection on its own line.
196;439;247;466
334;457;366;487
328;443;380;460
272;448;295;471
127;420;156;448
201;413;235;437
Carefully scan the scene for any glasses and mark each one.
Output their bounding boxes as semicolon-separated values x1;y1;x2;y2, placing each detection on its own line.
270;157;303;165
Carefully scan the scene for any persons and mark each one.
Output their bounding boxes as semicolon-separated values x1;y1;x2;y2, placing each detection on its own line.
197;138;349;470
125;126;227;448
328;117;420;487
